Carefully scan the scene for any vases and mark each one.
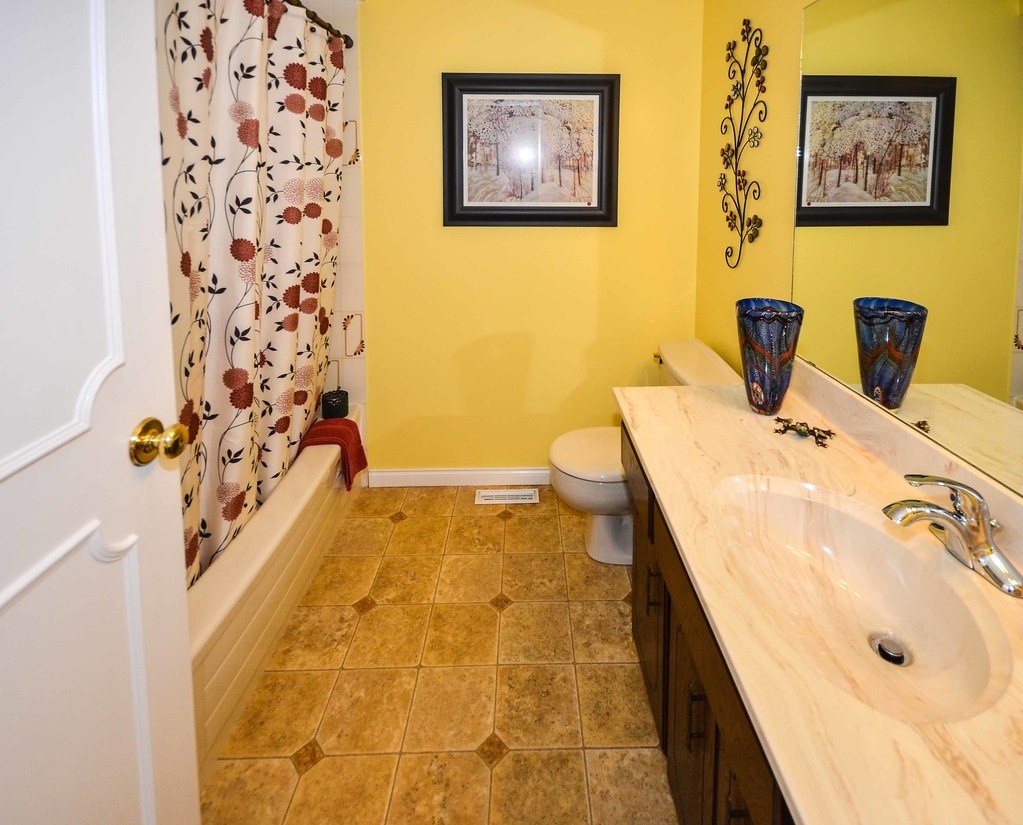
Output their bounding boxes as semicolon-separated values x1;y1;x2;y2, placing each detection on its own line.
852;296;928;411
735;298;805;416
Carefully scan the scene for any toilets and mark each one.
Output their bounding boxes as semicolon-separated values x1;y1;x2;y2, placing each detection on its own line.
548;336;744;567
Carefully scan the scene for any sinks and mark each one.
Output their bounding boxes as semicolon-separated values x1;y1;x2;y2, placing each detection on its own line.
714;473;1016;728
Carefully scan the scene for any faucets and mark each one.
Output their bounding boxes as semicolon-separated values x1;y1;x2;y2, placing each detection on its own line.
881;473;1023;600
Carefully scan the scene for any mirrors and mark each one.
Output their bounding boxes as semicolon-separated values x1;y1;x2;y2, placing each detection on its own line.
791;0;1023;496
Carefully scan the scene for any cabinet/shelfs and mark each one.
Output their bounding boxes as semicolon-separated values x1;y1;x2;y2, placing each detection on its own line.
620;421;794;825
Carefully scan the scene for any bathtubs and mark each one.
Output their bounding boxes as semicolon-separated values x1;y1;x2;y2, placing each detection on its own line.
184;401;363;794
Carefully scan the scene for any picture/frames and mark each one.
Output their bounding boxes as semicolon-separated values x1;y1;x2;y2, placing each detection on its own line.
441;71;621;229
795;74;958;227
